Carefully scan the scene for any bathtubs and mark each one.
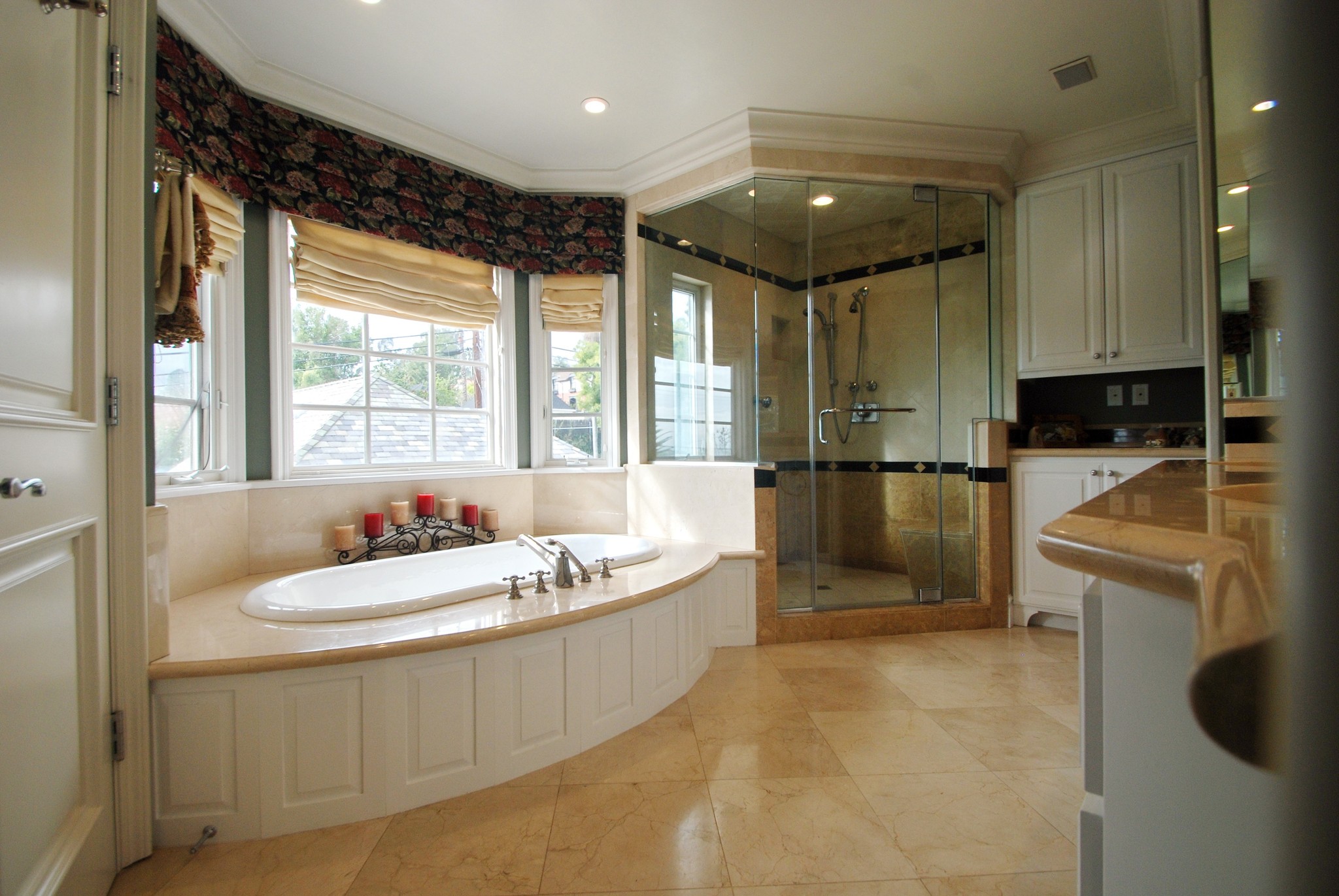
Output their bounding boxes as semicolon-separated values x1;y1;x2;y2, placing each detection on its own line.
238;531;662;621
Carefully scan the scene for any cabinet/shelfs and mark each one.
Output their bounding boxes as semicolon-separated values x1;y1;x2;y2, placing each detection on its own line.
1010;457;1207;634
1078;574;1292;894
1017;138;1200;377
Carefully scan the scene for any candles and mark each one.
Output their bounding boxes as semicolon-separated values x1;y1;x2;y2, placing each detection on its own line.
390;500;410;525
440;496;458;520
417;494;435;516
463;502;479;525
363;511;384;537
481;508;500;529
334;522;356;551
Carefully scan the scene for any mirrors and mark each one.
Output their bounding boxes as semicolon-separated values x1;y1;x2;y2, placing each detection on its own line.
1211;0;1292;627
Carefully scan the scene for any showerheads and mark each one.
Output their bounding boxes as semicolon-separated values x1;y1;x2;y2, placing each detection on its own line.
849;286;870;313
803;308;826;325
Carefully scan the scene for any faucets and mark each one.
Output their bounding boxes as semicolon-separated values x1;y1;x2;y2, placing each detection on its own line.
544;538;591;583
516;533;576;589
